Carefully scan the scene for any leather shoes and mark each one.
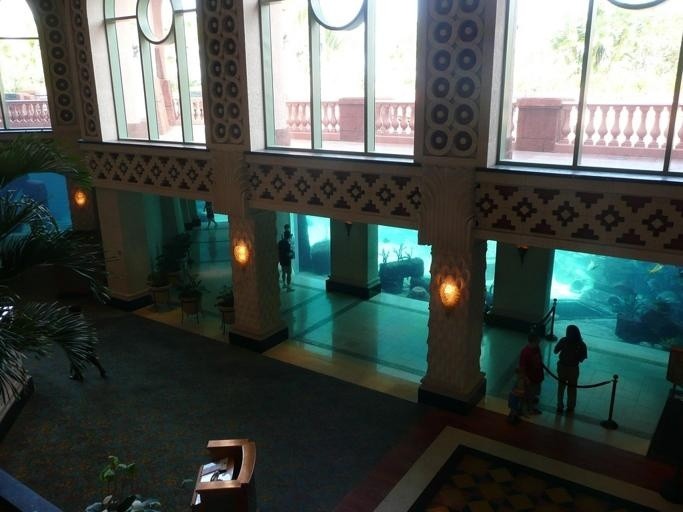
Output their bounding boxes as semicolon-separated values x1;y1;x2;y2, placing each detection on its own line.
69;373;83;380
556;407;563;414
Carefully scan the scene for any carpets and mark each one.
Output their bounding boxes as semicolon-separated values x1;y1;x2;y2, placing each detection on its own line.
0;301;429;512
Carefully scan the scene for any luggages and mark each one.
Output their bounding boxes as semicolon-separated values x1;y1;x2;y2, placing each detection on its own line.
507;378;532;419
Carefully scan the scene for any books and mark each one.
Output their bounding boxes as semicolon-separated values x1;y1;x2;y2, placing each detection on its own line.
193;454;233;507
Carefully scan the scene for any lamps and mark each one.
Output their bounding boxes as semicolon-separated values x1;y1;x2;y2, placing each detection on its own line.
75;189;87;207
439;273;460;317
233;239;250;265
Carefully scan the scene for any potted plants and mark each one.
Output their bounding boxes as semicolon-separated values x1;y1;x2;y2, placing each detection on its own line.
145;231;235;335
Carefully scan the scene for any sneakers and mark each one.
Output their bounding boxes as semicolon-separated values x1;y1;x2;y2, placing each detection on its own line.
530;409;540;414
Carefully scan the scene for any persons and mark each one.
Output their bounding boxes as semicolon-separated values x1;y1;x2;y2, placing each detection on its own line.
277;230;296;293
519;332;545;415
281;224;296;258
201;201;219;229
552;324;588;415
